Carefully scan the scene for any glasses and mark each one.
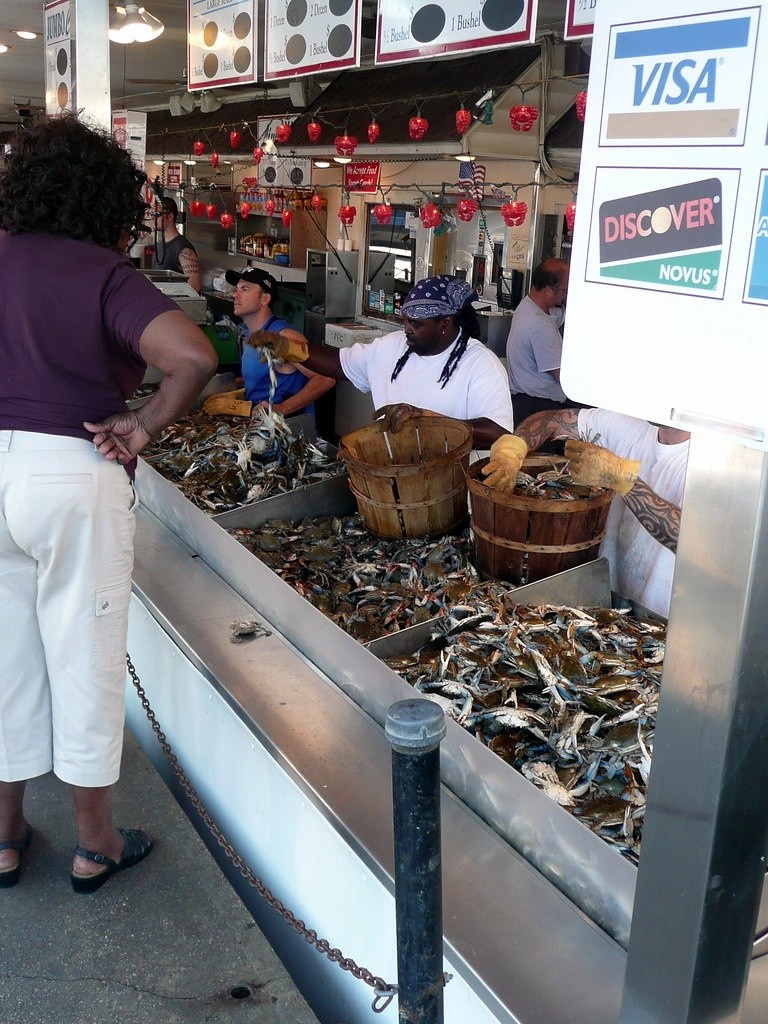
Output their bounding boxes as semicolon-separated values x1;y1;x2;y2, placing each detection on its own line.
150;211;170;217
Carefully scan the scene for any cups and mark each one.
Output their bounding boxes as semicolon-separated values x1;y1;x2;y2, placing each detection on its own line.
336;239;353;252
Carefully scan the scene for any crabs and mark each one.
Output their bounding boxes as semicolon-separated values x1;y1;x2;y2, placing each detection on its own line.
149;400;664;867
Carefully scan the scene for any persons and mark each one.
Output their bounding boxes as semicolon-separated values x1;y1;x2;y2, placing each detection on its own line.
0;108;219;894
482;406;691;625
504;259;572;455
246;275;514;541
203;268;336;435
149;196;201;294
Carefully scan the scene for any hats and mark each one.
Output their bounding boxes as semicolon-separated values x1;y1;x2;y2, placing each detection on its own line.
225;268;276;296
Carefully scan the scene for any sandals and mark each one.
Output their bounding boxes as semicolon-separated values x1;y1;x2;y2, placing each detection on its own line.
0;822;32;889
71;826;152;893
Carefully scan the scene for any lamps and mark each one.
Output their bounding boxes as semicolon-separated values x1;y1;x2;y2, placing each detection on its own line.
109;0;165;44
169;91;221;117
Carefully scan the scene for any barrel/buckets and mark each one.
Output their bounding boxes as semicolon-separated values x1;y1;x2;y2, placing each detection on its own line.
338;416;474;543
465;452;616;590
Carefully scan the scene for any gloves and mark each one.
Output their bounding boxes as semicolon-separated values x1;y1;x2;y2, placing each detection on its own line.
564;440;640;497
247;330;310;363
481;435;529;495
202;387;245;405
202;398;252;417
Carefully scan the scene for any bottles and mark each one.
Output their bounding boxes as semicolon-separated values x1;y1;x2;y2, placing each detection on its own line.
240;233;289;260
239;187;320;213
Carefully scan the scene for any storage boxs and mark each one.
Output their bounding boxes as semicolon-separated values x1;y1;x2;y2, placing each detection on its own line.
325;323;383;349
